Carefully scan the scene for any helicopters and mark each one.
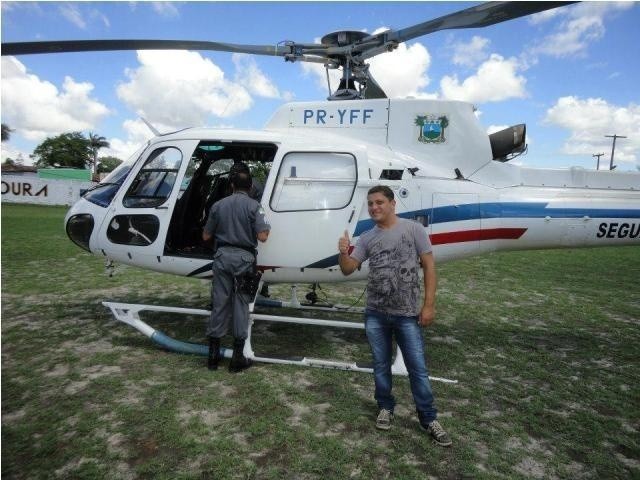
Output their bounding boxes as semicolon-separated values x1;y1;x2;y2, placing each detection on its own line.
2;1;640;383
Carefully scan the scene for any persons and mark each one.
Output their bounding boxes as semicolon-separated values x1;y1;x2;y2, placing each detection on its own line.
338;185;452;447
203;172;271;373
225;163;263;203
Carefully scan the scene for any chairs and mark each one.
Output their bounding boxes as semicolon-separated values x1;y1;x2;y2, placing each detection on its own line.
152;174;234;244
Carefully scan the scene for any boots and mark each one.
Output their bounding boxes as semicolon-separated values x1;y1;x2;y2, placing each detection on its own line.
229;341;252;372
208;337;221;370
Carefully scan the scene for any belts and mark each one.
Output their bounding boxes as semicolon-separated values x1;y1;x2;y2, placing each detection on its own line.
216;243;257;257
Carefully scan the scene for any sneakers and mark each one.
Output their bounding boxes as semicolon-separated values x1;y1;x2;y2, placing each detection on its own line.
376;405;395;430
418;420;453;447
